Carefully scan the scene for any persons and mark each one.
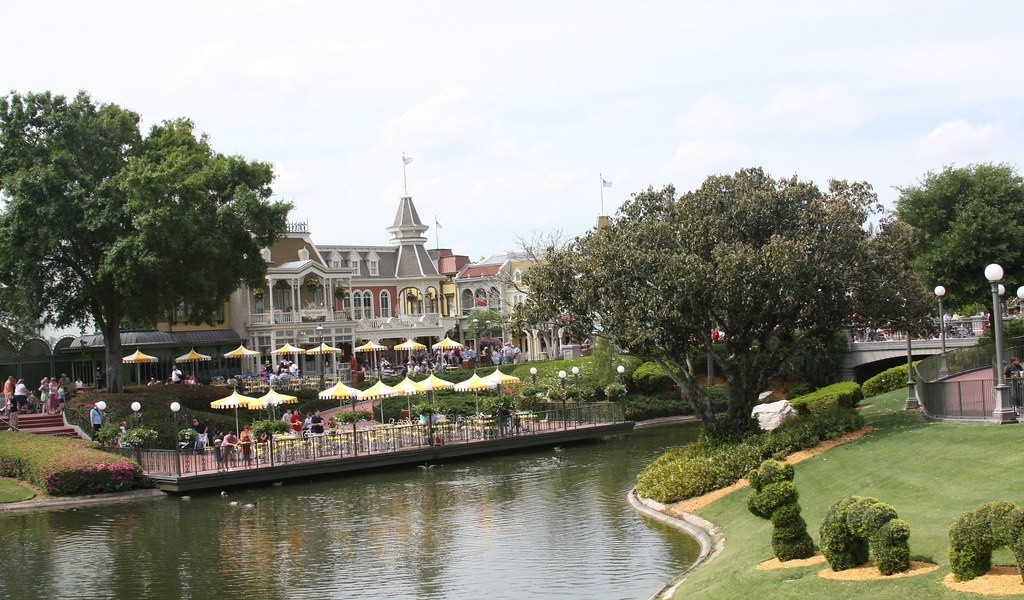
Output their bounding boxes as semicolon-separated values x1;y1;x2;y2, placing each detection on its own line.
4;373;84;416
401;348;435;375
350;354;369;372
192;416;253;469
483;342;521;365
257;359;299;386
168;366;199;384
117;421;132;447
95;367;106;389
434;347;475;365
90;405;102;440
380;357;393;378
283;407;327;447
146;377;156;385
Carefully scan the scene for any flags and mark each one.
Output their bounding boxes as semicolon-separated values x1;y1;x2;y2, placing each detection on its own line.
403;156;413;166
436;222;442;228
603;181;613;187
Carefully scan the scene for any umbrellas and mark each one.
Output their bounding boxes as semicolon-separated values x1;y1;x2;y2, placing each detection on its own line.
354;340;388;368
394;338;426;362
319;368;519;428
224;344;260;376
122;348;158;384
432;337;464;363
270;342;341;374
210;386;297;438
175;348;211;381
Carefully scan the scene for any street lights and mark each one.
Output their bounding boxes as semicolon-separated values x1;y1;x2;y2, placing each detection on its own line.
984;263;1019;424
558;370;567;429
315;324;326;391
934;285;950;380
131;402;141;464
572;366;582;425
95;400;107;449
616;366;627;423
170;401;182;477
901;298;918;409
473;317;480;368
485;320;491;357
529;367;539;423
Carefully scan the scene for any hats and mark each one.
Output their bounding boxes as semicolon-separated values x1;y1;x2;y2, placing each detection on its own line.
95;402;99;406
